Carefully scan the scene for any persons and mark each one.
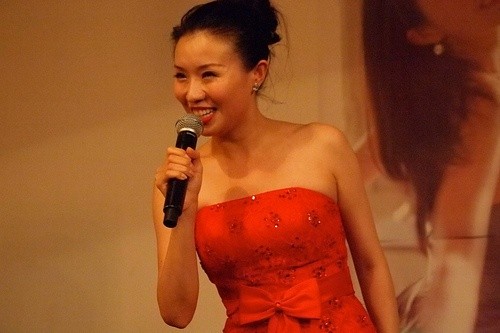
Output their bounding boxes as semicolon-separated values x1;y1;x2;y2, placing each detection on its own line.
361;0;499;332
152;0;400;333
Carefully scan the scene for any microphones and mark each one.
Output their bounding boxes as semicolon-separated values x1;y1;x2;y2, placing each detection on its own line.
163;113;204;228
